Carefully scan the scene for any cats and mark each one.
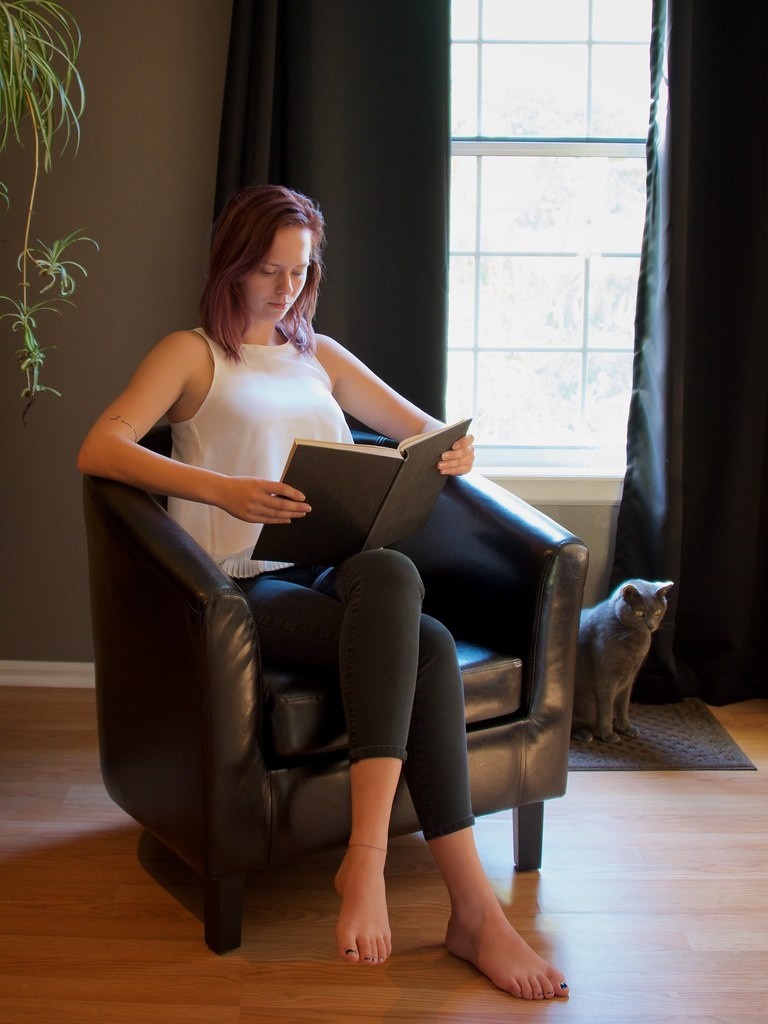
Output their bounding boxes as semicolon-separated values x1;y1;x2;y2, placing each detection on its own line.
572;578;674;744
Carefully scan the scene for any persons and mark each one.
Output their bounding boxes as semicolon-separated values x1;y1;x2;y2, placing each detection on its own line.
78;183;570;1002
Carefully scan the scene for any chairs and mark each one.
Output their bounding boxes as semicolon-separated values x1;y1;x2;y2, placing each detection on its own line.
80;430;590;952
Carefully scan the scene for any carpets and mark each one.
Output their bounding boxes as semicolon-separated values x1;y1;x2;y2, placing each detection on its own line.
567;697;756;771
